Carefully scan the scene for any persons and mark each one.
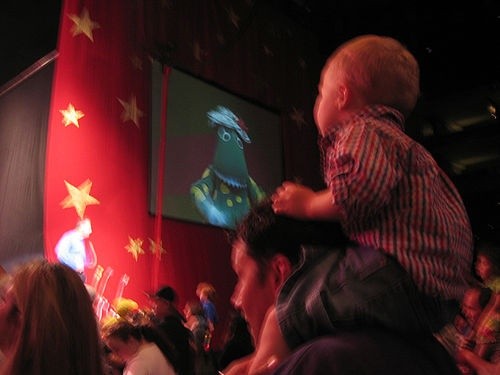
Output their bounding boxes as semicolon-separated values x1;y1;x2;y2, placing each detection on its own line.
93;283;256;374
241;34;472;374
448;249;500;375
0;252;118;375
228;199;464;375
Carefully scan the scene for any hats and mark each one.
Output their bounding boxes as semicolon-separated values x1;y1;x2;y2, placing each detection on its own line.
151;287;178;302
